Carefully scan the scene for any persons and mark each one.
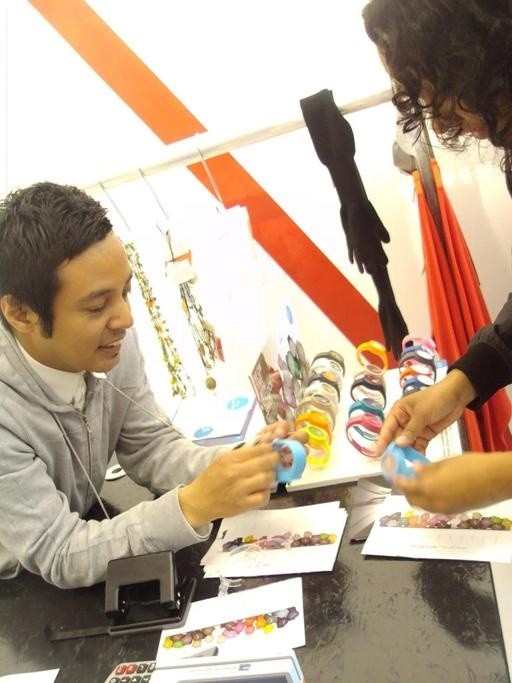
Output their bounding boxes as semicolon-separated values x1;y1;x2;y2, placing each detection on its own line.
0;182;309;590
362;0;512;515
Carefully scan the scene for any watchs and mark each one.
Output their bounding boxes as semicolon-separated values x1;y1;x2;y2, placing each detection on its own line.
380;441;432;485
290;333;437;470
270;438;307;483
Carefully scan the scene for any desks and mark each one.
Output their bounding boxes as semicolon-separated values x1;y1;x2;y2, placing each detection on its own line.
1;360;512;683
